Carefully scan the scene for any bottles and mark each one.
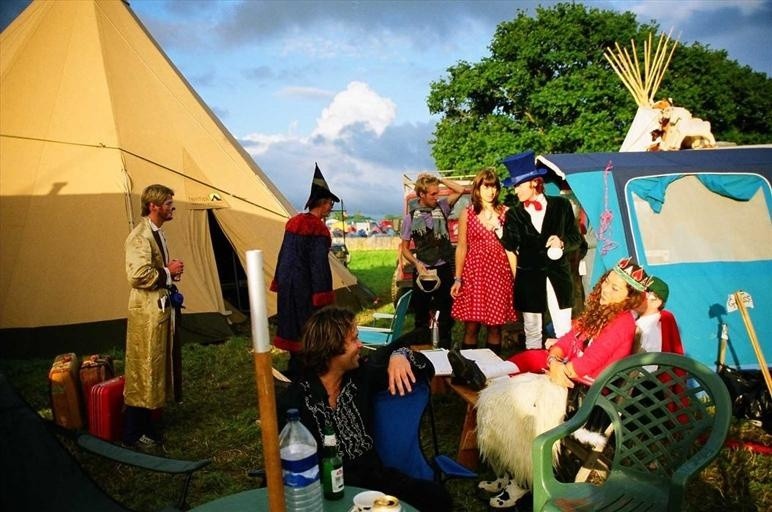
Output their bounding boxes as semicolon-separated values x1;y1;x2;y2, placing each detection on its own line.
278;409;324;512
320;427;346;502
371;494;402;512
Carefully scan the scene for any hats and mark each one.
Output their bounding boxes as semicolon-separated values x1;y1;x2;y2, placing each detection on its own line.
304;163;340;210
647;277;669;301
500;151;548;187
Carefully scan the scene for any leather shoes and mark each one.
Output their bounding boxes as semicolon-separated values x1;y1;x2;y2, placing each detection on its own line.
448;350;484;390
451;341;476;379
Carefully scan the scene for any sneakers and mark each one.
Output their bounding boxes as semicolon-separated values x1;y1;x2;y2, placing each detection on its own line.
477;473;512;493
490;481;532;507
122;434;158;448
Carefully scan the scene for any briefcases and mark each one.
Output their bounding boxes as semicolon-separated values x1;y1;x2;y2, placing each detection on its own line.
49;353;84;433
87;374;125;442
80;354;116;390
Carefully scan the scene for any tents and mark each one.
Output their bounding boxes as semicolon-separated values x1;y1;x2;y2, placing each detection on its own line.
535;144;770;374
1;0;379;353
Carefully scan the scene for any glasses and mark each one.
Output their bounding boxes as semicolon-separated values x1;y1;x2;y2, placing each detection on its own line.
323;199;334;206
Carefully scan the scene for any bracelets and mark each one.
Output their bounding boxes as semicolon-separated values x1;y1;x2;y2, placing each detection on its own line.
453;276;462;282
547;354;560;364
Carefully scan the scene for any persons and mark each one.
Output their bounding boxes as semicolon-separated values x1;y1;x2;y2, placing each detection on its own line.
446;274;667;390
275;306;453;511
121;185;185;451
474;263;648;508
397;173;465;349
272;198;340;369
449;170;517;354
483;177;583;351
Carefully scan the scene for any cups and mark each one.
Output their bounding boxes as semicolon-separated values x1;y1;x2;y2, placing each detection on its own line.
352;490;386;511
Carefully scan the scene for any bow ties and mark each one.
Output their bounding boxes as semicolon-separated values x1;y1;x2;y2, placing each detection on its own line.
525;200;541;210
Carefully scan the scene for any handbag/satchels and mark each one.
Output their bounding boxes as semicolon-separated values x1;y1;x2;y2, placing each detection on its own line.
404;262;455;314
